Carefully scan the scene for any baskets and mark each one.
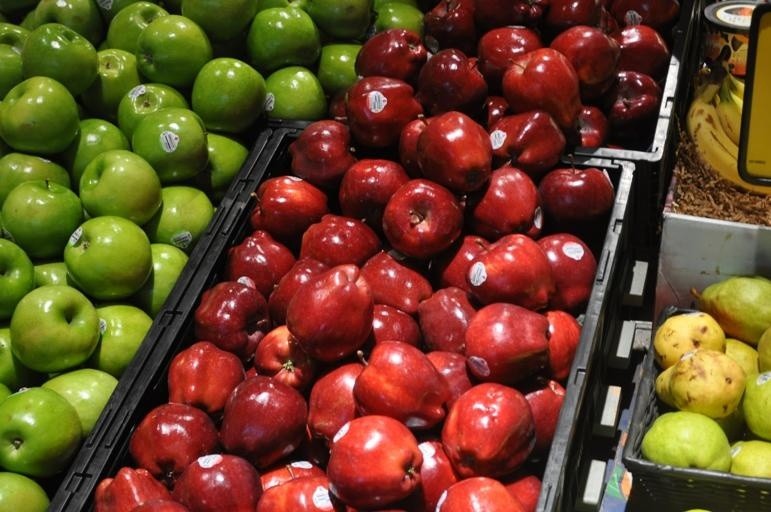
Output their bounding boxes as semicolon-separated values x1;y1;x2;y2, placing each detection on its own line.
621;303;771;512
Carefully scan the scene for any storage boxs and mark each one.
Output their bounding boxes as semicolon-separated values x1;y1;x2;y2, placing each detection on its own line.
654;150;771;320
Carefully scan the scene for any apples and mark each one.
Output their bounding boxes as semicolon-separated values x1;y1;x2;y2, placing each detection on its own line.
0;0;684;512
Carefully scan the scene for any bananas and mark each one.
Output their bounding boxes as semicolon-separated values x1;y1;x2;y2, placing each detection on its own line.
687;44;771;198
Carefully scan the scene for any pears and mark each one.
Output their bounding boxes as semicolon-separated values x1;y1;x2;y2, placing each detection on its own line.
640;276;771;481
